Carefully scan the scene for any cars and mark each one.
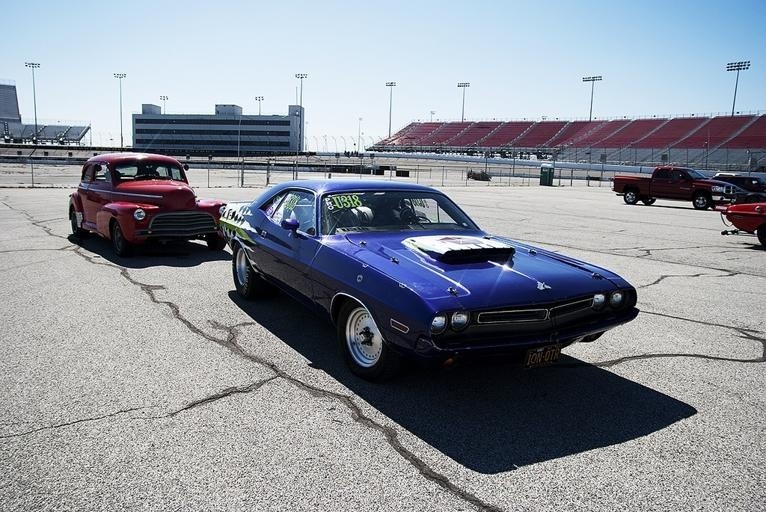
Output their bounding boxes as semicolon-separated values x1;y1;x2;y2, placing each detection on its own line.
217;176;641;377
68;151;230;259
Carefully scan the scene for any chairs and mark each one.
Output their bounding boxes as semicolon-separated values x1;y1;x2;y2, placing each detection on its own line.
326;206;404;235
105;171;120;182
136;169;158;178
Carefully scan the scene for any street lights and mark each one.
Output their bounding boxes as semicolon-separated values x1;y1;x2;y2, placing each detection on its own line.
582;75;603;122
385;81;397;138
24;62;41;138
457;82;471;123
295;73;308;107
726;61;751;116
159;95;169;114
255;96;265;115
112;71;127;147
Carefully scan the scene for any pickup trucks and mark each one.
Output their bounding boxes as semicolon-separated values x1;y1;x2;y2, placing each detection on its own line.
613;165;738;211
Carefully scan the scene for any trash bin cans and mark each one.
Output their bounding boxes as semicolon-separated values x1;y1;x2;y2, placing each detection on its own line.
540;164;554;186
